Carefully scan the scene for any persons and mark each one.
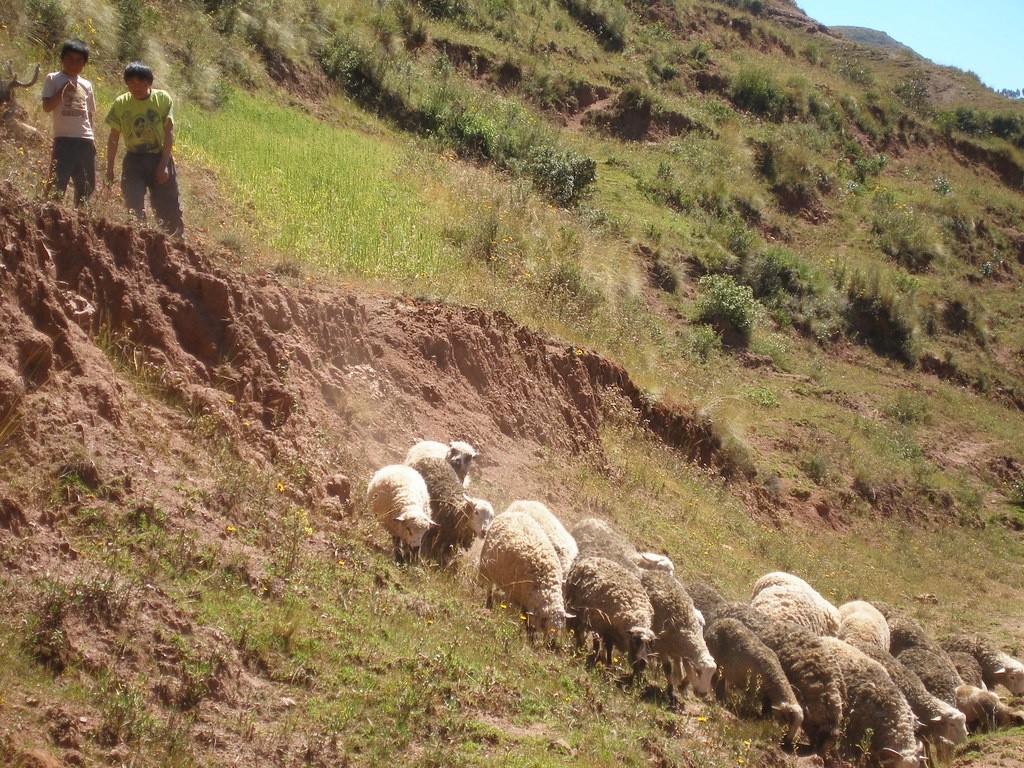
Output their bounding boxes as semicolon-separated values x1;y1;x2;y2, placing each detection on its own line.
40;41;97;215
104;61;186;248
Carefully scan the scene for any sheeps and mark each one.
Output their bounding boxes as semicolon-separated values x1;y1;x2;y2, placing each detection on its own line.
365;438;1024;768
